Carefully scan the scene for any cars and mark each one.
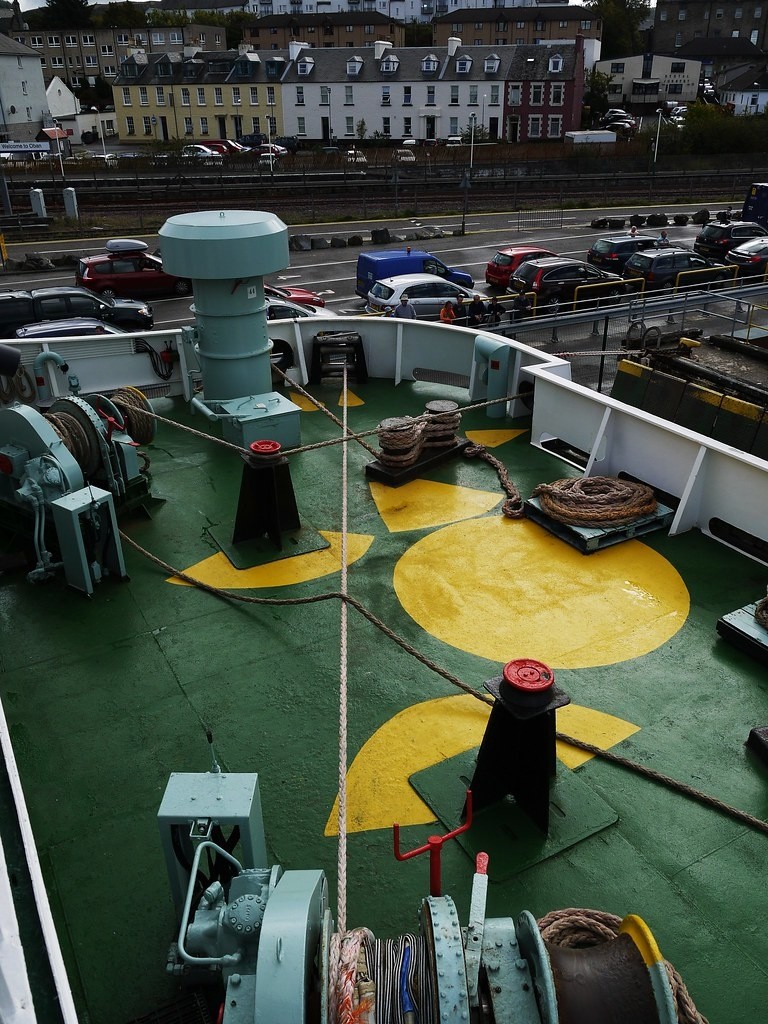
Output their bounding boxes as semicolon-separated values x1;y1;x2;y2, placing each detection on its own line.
0;136;343;167
506;256;626;315
366;273;490;322
423;138;439;147
596;78;715;143
587;233;667;275
445;137;466;147
263;282;325;309
725;236;768;283
623;244;730;296
391;149;415;163
14;315;126;340
347;150;368;165
76;238;194;303
485;246;559;290
264;295;339;322
403;140;419;148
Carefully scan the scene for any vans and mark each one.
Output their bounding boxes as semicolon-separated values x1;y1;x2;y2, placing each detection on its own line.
354;245;475;302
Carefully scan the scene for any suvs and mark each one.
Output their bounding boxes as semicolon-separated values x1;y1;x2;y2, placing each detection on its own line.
692;219;768;261
0;285;154;340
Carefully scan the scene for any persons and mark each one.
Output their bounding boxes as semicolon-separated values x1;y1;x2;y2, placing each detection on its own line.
513;290;531;323
487;294;507;327
469;295;487;329
626;205;732;248
440;301;456;324
394;294;417;319
453;294;467;327
381;306;395;317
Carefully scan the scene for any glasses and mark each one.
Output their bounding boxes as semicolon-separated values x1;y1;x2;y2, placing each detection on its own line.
520;293;525;295
494;299;498;301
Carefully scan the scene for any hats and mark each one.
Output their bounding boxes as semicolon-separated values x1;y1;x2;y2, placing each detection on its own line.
492;296;498;300
400;294;409;301
384;306;392;311
518;289;525;295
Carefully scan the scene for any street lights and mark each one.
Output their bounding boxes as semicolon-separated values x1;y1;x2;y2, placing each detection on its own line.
649;107;664;186
753;82;759;117
91;107;106;155
52;119;65;190
236;98;242;140
482;94;487;143
468;111;476;181
265;114;273;187
327;86;332;147
